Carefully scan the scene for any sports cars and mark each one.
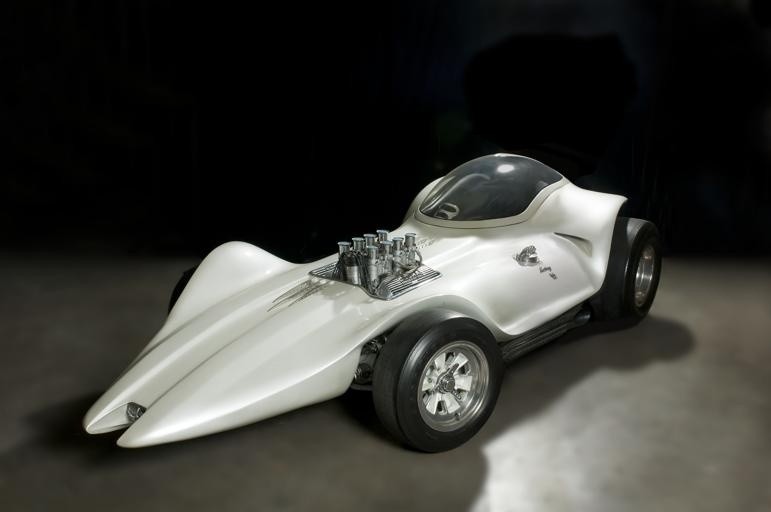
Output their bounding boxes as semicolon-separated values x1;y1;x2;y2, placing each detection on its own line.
81;152;663;456
